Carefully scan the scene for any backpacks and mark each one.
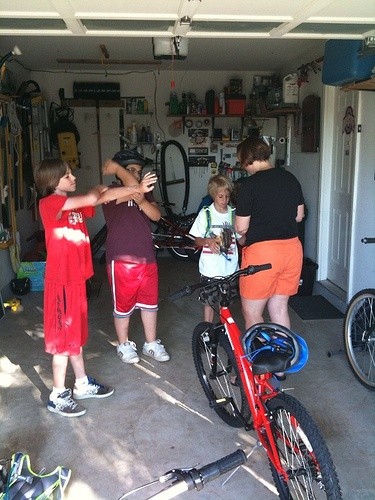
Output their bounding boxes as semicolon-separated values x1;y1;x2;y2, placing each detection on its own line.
0;452;72;500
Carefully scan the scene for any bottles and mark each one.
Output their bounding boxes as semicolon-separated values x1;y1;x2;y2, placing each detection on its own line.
124;96;154;148
169;90;226;115
248;72;299;116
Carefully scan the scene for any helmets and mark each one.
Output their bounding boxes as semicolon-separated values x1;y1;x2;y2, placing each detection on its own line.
112;149;149;166
241;322;309;373
10;277;31;296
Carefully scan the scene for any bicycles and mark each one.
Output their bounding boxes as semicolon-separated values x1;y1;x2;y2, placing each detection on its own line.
343;235;375;390
69;447;247;499
163;261;346;499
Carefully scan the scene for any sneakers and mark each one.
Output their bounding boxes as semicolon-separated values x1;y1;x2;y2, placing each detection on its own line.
73;376;115;399
142;339;169;362
47;387;86;417
116;340;139;363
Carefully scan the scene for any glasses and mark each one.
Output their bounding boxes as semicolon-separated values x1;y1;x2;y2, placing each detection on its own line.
127;167;142;177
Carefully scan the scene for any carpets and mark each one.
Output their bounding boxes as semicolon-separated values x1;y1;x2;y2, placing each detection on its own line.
288;295;347;320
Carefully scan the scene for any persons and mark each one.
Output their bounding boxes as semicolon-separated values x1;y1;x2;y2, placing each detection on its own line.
101;150;170;363
230;136;304;387
188;175;246;323
33;159;158;417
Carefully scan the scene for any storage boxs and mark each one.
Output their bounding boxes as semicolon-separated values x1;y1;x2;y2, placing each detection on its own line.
16;252;48;292
225;100;246;115
323;39;375;87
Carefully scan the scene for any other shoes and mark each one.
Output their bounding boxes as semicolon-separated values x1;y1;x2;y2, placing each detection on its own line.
273;373;286;380
201;332;211;352
230;375;240;386
